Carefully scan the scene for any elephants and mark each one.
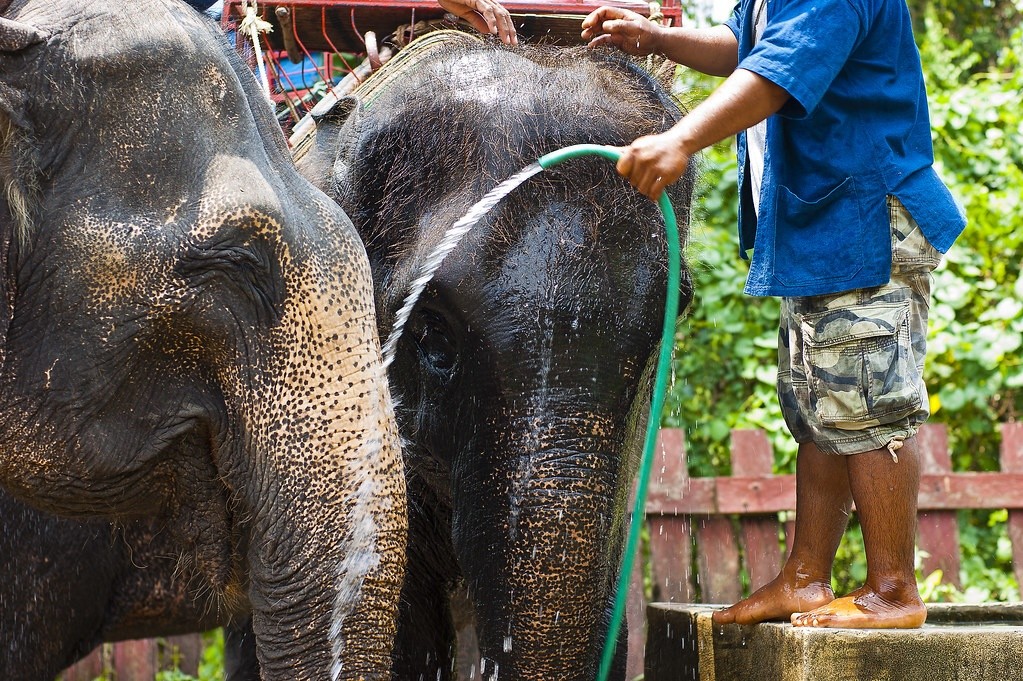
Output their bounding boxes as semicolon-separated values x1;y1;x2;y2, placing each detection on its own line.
0;0;704;681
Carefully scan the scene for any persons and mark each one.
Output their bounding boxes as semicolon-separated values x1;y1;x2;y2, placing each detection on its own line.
580;0;966;630
